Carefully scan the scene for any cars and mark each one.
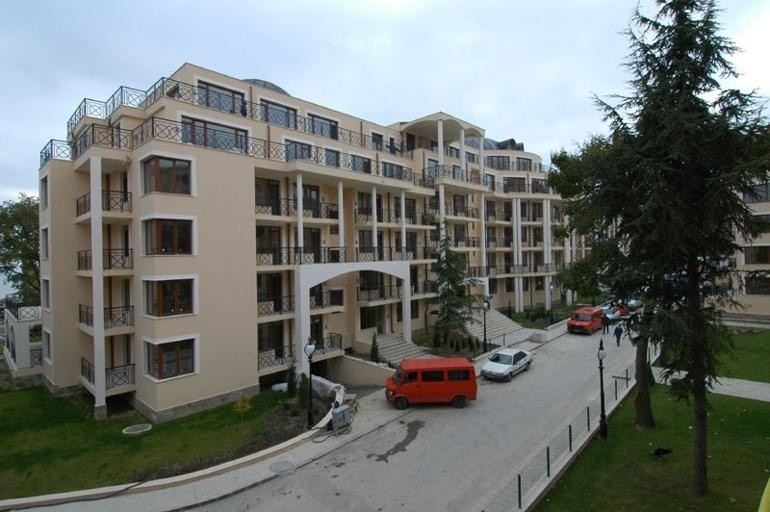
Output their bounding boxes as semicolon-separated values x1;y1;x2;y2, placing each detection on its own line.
480;348;534;383
599;295;644;324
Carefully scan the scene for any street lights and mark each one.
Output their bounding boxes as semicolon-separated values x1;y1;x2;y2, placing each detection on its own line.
597;339;610;440
303;336;319;430
481;297;491;354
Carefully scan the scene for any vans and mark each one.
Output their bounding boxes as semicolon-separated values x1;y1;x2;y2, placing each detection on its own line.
567;305;604;335
384;356;478;409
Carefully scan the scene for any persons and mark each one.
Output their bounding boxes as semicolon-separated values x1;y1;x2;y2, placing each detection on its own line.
601;313;612;334
613;324;623;346
